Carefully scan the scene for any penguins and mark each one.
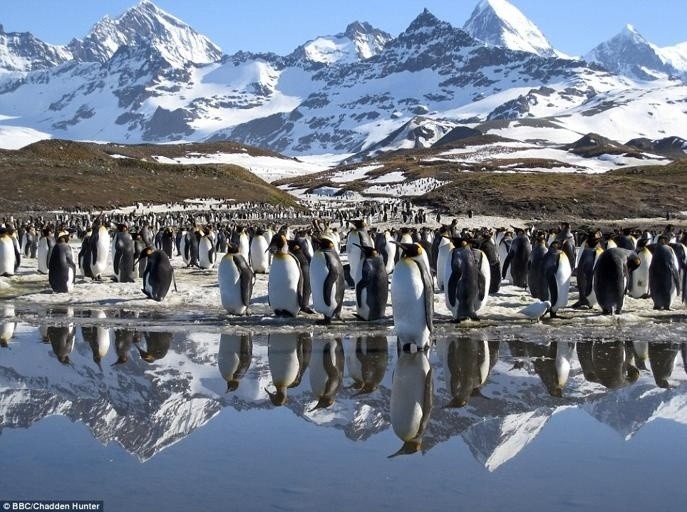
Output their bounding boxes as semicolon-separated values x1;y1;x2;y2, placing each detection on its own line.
0;303;687;459
0;199;687;352
